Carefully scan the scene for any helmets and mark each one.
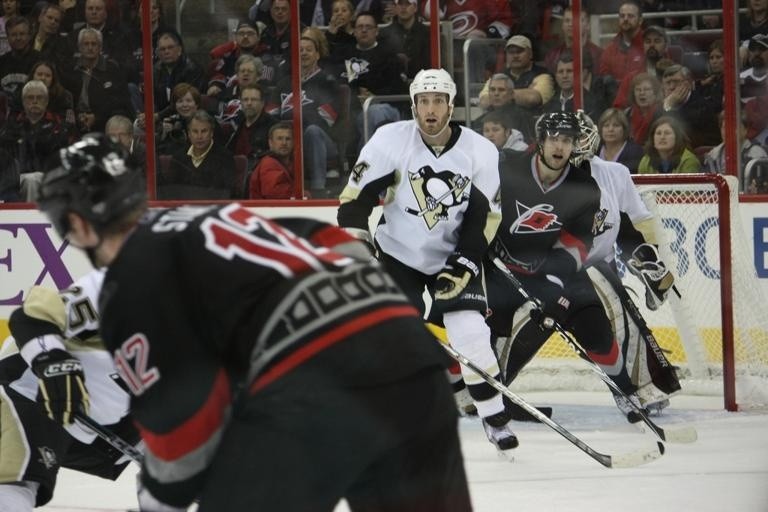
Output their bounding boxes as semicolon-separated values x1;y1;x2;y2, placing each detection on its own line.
572;109;601;157
35;130;150;234
534;112;581;157
409;68;457;109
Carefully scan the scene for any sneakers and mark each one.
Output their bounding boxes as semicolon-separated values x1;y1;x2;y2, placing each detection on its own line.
482;418;519;452
613;393;647;424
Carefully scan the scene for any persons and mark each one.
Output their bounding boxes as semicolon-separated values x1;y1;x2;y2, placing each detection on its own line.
476;4;767;191
0;0;430;205
337;69;519;449
0;265;143;508
480;111;651;421
450;108;681;417
422;0;512;60
36;130;472;511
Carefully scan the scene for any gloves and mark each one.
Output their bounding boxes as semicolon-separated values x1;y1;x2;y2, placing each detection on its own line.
529;293;571;342
31;348;91;427
433;256;481;303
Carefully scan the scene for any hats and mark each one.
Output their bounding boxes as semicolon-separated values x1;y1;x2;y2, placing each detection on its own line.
748;33;768;50
503;35;533;51
236;19;258;34
642;25;666;40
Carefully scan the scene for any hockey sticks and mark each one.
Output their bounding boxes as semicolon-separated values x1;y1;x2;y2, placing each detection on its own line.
435;338;664;468
486;248;696;443
